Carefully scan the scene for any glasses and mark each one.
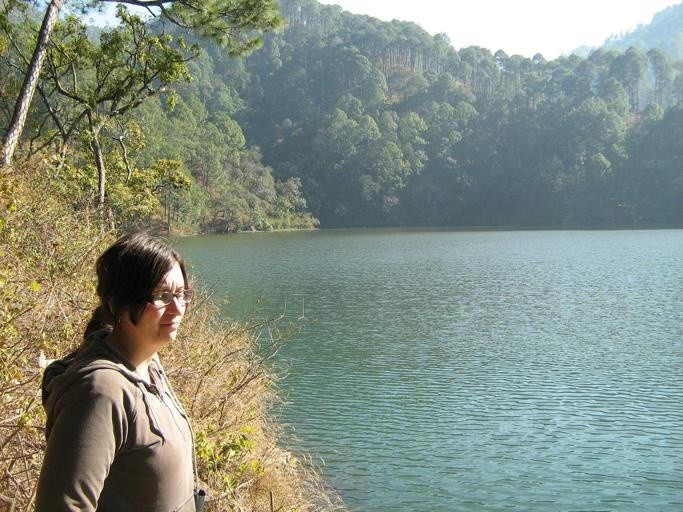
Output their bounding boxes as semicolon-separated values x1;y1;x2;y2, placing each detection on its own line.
147;288;194;307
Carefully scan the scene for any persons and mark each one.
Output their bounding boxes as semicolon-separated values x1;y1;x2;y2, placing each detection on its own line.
35;233;197;511
